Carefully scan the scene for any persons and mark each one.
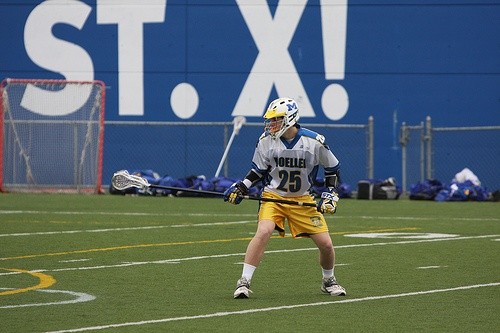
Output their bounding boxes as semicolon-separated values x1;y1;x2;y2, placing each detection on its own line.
223;98;347;299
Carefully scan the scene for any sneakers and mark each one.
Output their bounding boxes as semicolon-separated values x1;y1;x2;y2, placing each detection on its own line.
234;278;254;299
320;276;346;296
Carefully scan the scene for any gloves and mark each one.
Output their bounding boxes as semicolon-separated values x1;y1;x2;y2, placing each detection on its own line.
317;188;339;214
223;180;250;205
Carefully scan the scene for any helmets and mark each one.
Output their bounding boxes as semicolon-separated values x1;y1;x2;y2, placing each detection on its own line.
263;97;300;139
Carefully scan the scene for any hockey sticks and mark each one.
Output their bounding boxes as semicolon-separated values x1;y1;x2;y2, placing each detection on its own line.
215;116;246;178
111;170;318;207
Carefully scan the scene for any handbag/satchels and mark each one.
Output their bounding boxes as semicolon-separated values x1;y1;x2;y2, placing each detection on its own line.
357;177;402;200
